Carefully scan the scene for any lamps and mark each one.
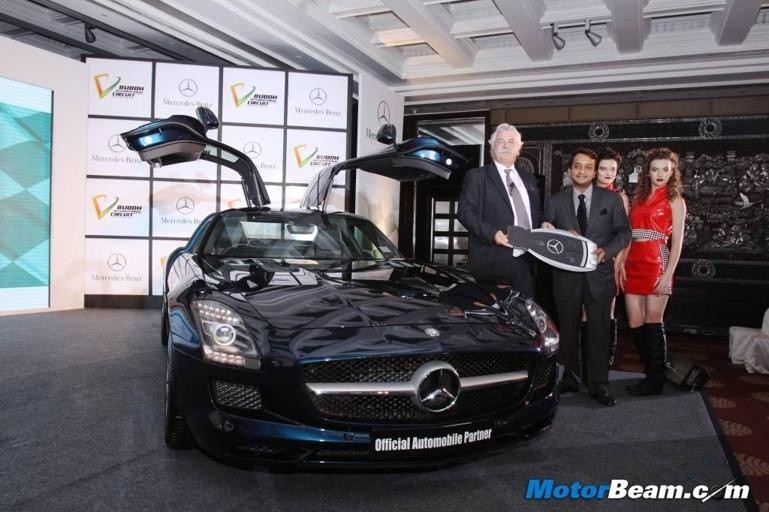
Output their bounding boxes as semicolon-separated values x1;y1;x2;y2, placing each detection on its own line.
550;23;565;50
584;19;602;46
85;23;96;42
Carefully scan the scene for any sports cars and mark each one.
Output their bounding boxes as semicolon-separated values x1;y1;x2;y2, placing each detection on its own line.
119;106;559;473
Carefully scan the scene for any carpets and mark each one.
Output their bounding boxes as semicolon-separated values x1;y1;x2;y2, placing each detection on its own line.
0;308;761;512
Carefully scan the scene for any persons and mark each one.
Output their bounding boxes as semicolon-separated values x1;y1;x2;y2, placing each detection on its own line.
544;148;632;408
577;149;630;386
618;146;688;396
456;122;555;305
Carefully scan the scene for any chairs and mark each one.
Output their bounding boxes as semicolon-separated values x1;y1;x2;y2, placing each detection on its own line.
212;221;351;259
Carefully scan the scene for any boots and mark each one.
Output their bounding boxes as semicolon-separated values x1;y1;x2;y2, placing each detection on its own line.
608;319;619;368
581;321;587;370
626;322;668;395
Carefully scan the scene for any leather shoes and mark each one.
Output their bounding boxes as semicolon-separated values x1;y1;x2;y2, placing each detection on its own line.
555;383;579;395
589;392;617;406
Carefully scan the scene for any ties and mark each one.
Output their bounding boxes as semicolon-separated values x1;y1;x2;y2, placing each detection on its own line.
577;194;587;233
504;170;530;231
